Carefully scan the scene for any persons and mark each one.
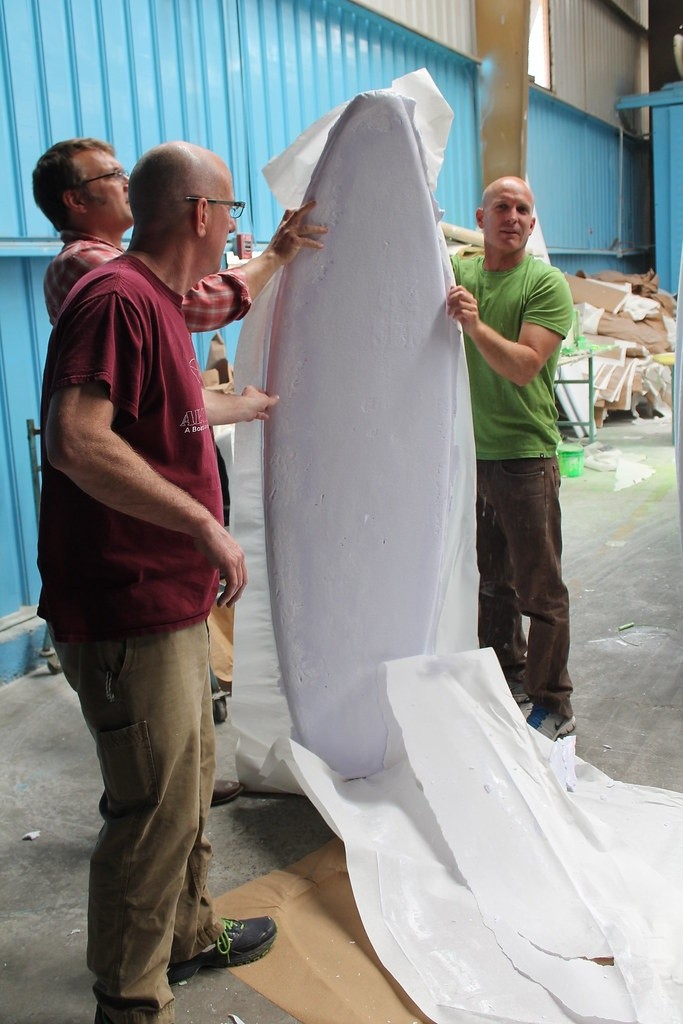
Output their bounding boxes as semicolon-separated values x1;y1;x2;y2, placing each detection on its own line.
19;141;281;1024
447;175;576;740
33;137;328;808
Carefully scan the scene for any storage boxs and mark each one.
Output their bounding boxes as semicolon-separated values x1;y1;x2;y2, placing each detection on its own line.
557;443;584;477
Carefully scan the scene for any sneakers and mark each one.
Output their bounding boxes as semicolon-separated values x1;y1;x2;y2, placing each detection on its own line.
158;916;278;988
527;705;576;744
508;676;533;703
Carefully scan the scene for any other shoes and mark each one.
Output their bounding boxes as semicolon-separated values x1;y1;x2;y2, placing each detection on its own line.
209;778;246;804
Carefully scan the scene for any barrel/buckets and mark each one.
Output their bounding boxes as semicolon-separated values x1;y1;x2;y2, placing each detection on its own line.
557;443;585;477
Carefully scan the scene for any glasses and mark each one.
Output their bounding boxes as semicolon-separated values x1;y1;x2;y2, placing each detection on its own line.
79;168;130;183
186;196;246;220
